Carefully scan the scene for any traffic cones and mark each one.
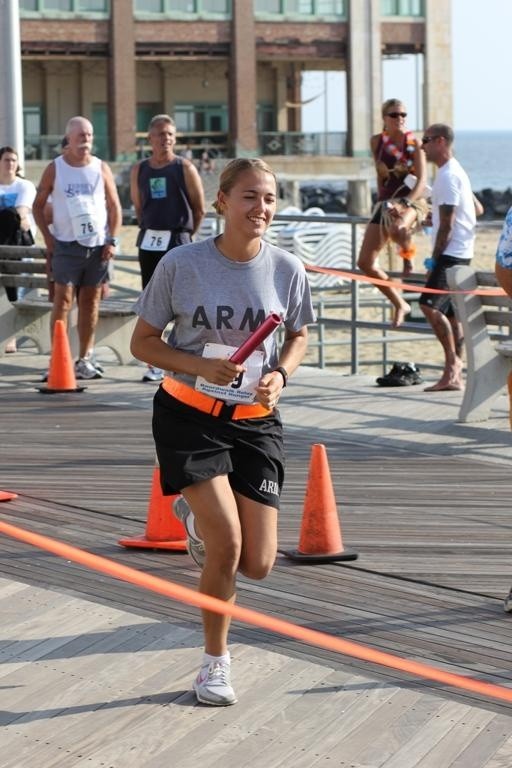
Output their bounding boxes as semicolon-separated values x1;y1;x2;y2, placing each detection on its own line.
119;445;192;556
34;318;88;394
278;440;360;564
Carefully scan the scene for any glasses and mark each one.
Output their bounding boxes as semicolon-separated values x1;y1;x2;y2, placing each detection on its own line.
386;111;406;117
423;137;437;143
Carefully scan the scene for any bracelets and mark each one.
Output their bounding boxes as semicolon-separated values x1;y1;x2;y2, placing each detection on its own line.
105;237;118;247
276;367;289;387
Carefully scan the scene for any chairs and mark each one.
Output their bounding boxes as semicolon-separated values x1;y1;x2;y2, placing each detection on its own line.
173;200;389;301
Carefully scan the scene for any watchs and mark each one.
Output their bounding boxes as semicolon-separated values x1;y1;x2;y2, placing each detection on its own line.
401;199;411;207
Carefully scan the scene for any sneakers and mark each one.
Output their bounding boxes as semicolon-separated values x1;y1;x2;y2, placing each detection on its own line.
193;661;248;706
172;495;209;568
74;355;104;379
375;361;423;386
141;367;166;382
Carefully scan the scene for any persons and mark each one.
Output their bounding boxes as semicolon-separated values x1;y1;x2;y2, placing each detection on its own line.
200;152;214;174
129;114;207;382
35;115;123;380
419;123;484;391
495;203;511;616
1;147;37;302
357;99;427;329
43;135;111;373
182;139;195;162
129;157;317;707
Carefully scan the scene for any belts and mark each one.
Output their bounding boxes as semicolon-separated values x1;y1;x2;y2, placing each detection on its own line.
161;375;273;420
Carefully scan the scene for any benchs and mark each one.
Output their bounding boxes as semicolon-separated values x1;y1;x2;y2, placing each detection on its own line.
443;265;512;424
1;246;145;366
